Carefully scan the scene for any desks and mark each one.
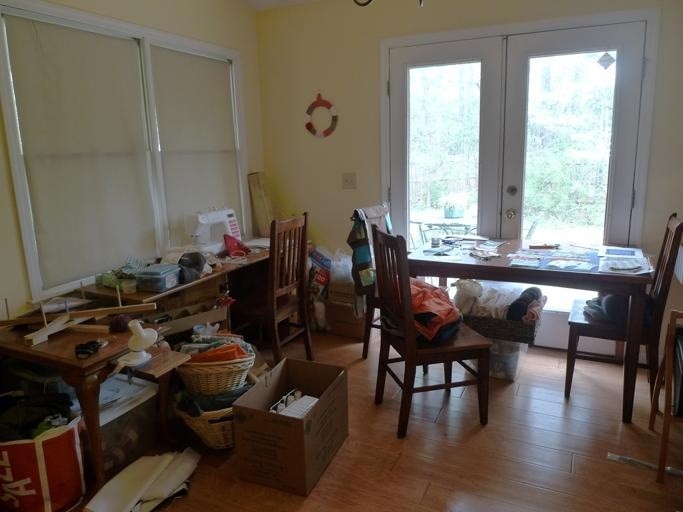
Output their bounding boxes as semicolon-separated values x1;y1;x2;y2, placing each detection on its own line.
0;301;172;495
77;237;312;316
406;237;653;425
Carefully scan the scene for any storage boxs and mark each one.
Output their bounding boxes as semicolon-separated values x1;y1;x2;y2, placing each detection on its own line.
326;300;365;341
468;337;528;384
232;357;349;498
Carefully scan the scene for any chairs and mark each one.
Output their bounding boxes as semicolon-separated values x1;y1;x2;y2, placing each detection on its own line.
371;224;493;440
233;211;315;362
354;203;398;360
564;212;682;424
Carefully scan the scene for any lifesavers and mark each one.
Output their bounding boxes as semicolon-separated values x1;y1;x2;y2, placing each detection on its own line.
304;100;338;138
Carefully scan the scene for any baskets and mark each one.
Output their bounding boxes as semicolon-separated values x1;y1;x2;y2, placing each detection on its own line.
172;390;235;453
174;346;256;395
